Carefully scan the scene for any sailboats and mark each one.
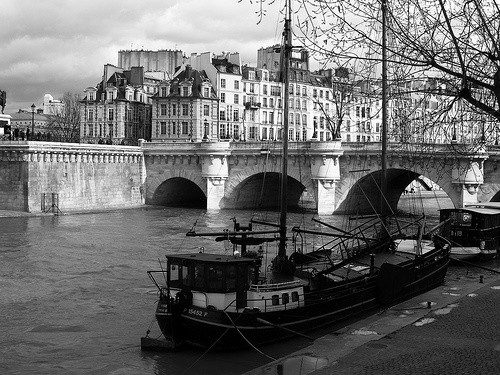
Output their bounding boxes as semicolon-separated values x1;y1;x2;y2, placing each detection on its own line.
142;3;456;354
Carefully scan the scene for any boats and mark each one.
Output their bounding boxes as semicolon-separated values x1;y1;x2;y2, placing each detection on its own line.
433;201;500;259
